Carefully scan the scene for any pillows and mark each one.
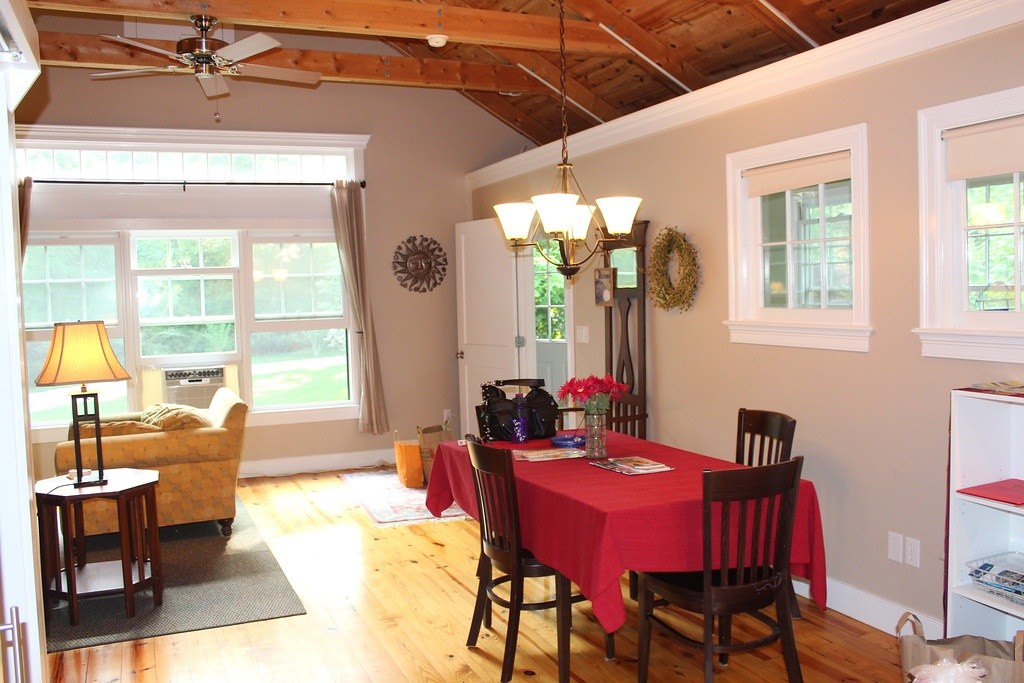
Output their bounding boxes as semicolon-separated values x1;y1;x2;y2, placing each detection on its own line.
142;401;215;431
78;420;164;439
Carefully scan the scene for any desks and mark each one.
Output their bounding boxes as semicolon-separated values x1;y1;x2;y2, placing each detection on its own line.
425;426;827;662
33;466;161;626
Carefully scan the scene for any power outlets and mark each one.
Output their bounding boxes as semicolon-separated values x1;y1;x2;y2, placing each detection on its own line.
443;409;452;424
906;537;920;569
888;532;903;564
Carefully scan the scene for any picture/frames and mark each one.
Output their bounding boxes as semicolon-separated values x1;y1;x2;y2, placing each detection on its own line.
593;268;617;306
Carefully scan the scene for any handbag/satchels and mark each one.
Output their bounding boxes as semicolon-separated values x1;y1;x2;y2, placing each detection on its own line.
897;611;1024;683
394;430;424;488
475;384;560;441
417;419;453;484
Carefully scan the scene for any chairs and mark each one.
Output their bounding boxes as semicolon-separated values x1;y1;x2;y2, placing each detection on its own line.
466;378;805;683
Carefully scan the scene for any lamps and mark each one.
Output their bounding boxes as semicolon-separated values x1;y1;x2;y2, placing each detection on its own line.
970;185;1007;233
34;319;132;490
492;0;643;280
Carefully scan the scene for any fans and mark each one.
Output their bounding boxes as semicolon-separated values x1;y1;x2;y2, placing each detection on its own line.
87;14;323;118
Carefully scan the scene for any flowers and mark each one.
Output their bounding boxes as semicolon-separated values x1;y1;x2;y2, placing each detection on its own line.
559;372;629;415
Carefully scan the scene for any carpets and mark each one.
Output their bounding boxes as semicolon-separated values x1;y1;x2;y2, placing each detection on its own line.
43;486;308;655
335;465;474;530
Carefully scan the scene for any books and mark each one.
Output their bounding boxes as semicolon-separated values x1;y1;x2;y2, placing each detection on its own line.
513;447;586;462
589;456;675;475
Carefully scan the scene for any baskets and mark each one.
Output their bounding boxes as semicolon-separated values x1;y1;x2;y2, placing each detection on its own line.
964;551;1024;607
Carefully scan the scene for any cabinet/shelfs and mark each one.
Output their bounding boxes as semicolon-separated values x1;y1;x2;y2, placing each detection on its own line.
943;379;1024;640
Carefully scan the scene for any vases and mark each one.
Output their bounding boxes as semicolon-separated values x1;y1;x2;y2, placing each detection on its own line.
584;414;607;459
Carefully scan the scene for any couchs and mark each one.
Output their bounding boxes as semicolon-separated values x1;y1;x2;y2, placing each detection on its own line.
49;386;249;557
